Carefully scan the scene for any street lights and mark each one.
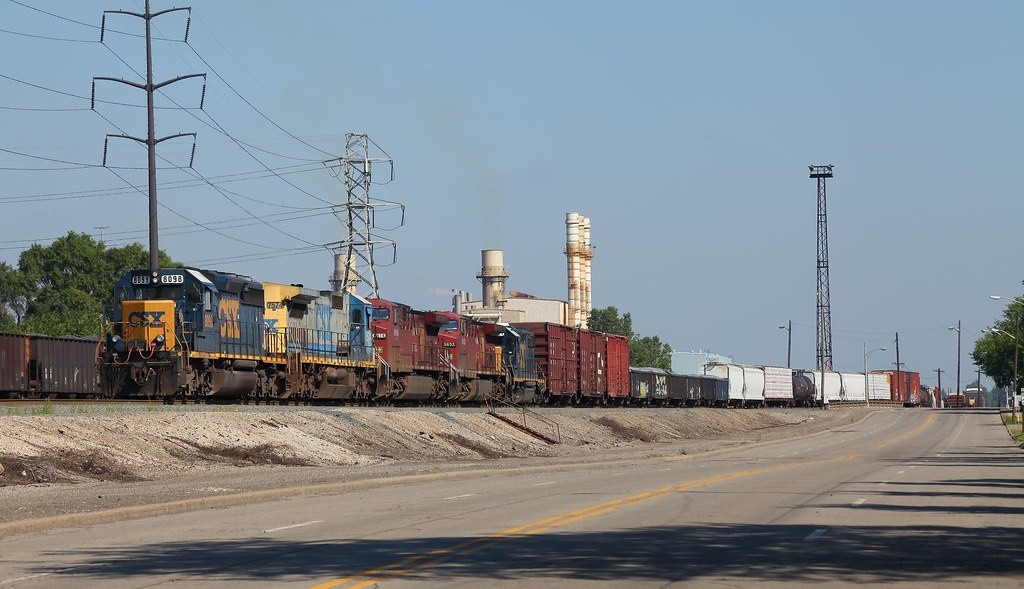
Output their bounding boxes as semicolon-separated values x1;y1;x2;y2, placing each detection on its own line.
865;347;886;407
989;295;1021;424
779;319;792;368
948;327;960;407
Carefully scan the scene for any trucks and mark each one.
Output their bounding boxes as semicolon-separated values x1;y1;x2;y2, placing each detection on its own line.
963;388;985;407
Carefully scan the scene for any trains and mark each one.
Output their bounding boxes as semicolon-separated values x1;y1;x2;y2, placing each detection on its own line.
92;266;942;408
0;332;105;400
